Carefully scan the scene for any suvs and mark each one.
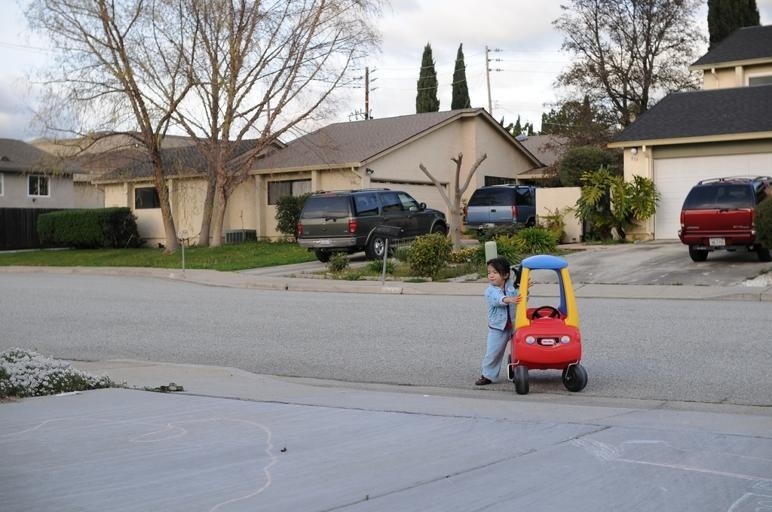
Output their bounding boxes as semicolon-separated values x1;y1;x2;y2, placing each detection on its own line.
296;187;449;261
464;183;535;243
678;175;772;263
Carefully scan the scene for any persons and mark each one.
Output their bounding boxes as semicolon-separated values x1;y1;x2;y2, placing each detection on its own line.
475;258;535;385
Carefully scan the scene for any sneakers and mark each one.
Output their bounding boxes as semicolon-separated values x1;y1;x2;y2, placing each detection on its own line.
475;375;491;385
